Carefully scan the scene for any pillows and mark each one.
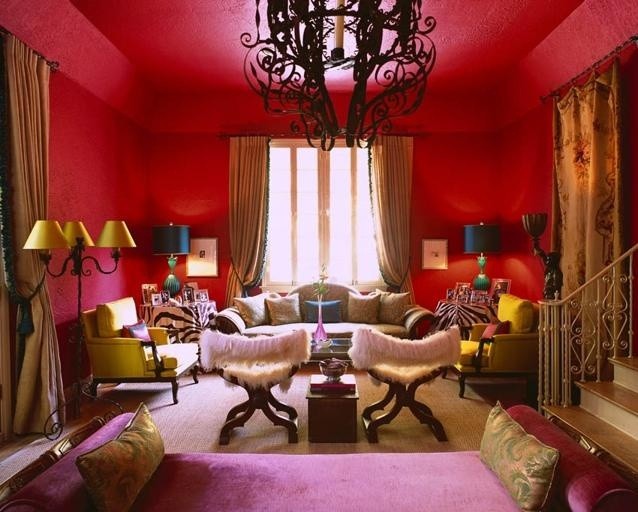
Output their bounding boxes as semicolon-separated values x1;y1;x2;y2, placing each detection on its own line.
233;291;280;328
347;291;381;323
498;294;533;332
376;288;410;326
481;320;510;345
266;293;302;326
479;399;561;512
96;297;137;337
368;292;376;296
75;401;165;512
122;319;150;347
304;300;341;323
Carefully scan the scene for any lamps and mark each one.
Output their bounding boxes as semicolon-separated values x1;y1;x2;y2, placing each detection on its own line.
22;219;137;440
151;222;191;298
240;1;436;151
464;222;498;290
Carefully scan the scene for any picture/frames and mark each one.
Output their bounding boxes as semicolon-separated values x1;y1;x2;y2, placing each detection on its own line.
421;239;448;270
455;282;470;289
185;237;218;277
141;282;209;306
490;278;511;304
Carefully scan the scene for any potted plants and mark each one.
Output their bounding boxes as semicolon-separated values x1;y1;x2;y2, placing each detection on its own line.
310;265;328;344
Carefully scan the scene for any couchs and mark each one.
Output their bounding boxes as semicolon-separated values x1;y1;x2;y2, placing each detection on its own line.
0;405;630;512
216;292;434;339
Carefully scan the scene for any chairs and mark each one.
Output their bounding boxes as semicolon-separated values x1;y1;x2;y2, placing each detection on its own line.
198;329;310;445
81;307;199;404
441;303;540;397
348;324;462;444
